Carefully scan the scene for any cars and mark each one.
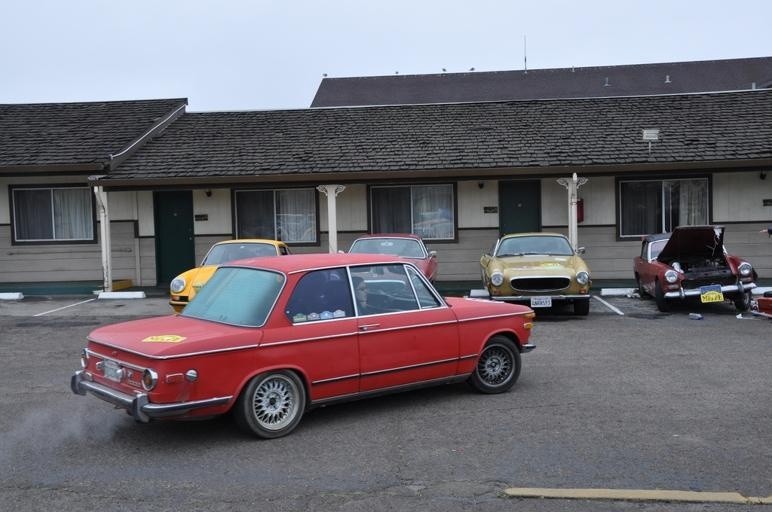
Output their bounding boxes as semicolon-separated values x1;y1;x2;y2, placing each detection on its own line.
335;232;442;290
167;238;300;321
631;222;760;316
67;251;538;444
478;230;594;317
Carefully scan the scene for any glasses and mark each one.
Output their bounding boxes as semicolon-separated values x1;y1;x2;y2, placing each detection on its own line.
356;284;368;291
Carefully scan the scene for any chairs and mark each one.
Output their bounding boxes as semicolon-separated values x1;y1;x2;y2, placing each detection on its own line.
301;272;361;317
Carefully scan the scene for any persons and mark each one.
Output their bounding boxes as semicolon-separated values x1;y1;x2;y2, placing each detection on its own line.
349;276;379;315
